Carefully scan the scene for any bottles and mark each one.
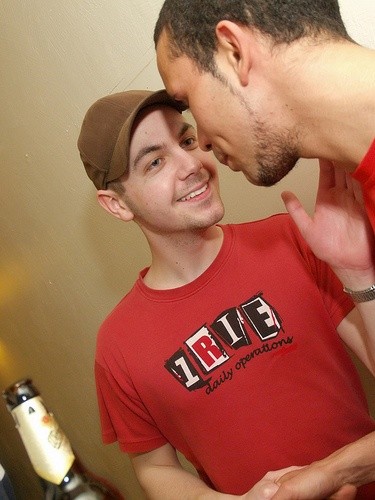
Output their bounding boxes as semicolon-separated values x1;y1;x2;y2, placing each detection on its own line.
2;376;126;500
0;463;17;500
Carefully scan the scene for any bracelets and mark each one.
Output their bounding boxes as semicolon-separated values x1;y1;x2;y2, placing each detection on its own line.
341;281;374;304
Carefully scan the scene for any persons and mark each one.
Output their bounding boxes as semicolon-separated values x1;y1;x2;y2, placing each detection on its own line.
151;0;375;500
74;88;374;500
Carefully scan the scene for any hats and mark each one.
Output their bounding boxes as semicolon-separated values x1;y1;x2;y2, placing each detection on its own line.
78;90;183;190
